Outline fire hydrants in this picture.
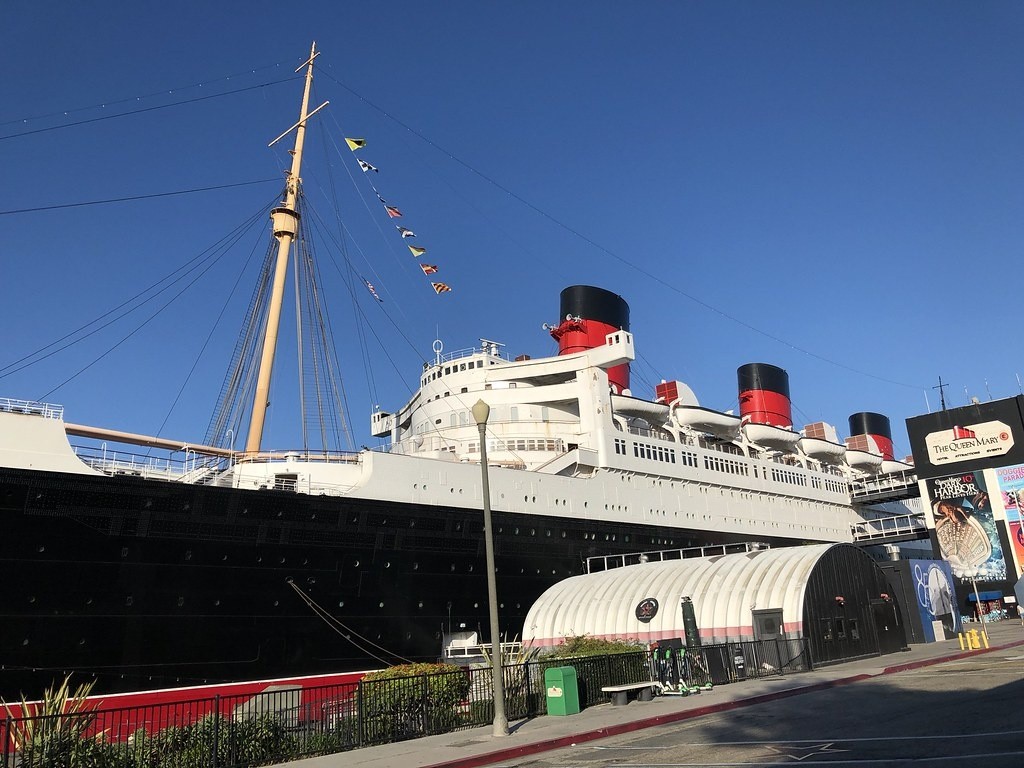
[966,628,981,649]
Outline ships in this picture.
[0,40,928,704]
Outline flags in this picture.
[357,160,378,173]
[374,196,451,295]
[344,138,366,151]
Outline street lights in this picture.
[956,566,990,639]
[471,400,510,738]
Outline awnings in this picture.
[969,591,1002,601]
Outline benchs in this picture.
[601,680,660,707]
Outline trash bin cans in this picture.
[545,666,580,716]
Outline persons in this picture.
[936,500,973,531]
[1017,605,1024,626]
